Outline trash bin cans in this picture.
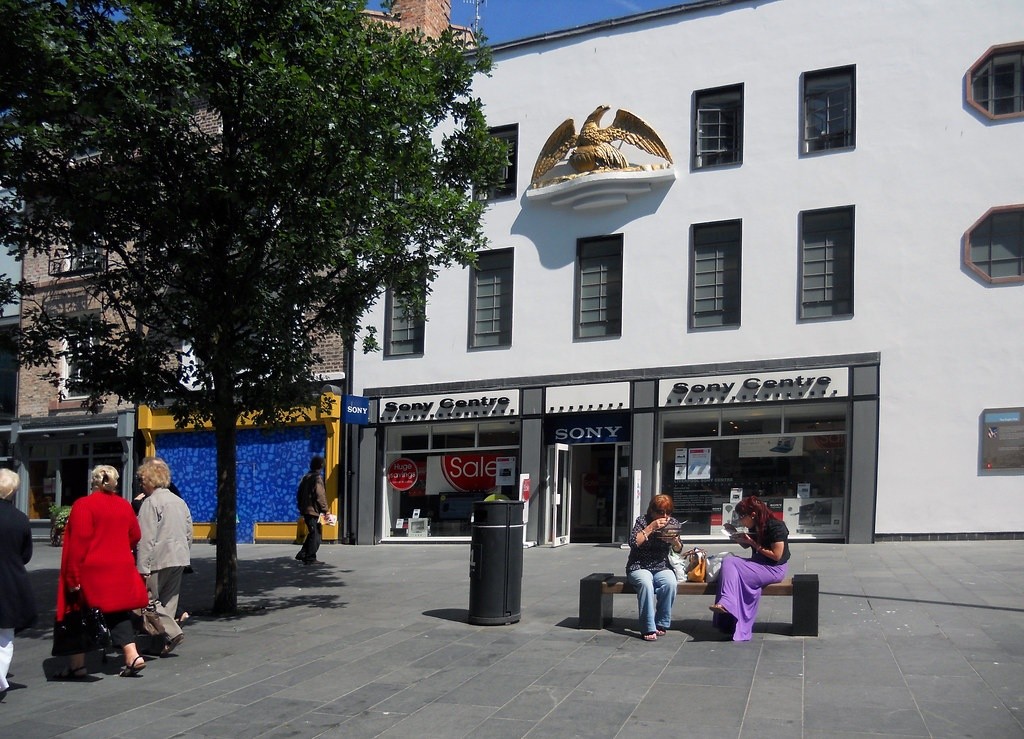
[468,500,523,625]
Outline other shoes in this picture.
[159,634,183,657]
[295,551,326,566]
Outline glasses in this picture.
[653,509,673,515]
[738,513,750,519]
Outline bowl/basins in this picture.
[733,533,756,540]
[661,524,682,537]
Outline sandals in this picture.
[175,611,191,630]
[118,656,146,678]
[656,625,666,636]
[52,666,88,681]
[641,632,658,641]
[709,603,729,614]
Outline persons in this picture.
[295,456,328,565]
[0,468,33,701]
[626,495,683,641]
[56,457,193,677]
[709,496,791,641]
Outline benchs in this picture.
[579,573,819,636]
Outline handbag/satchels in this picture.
[667,545,730,584]
[51,591,114,657]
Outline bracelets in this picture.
[642,530,648,541]
